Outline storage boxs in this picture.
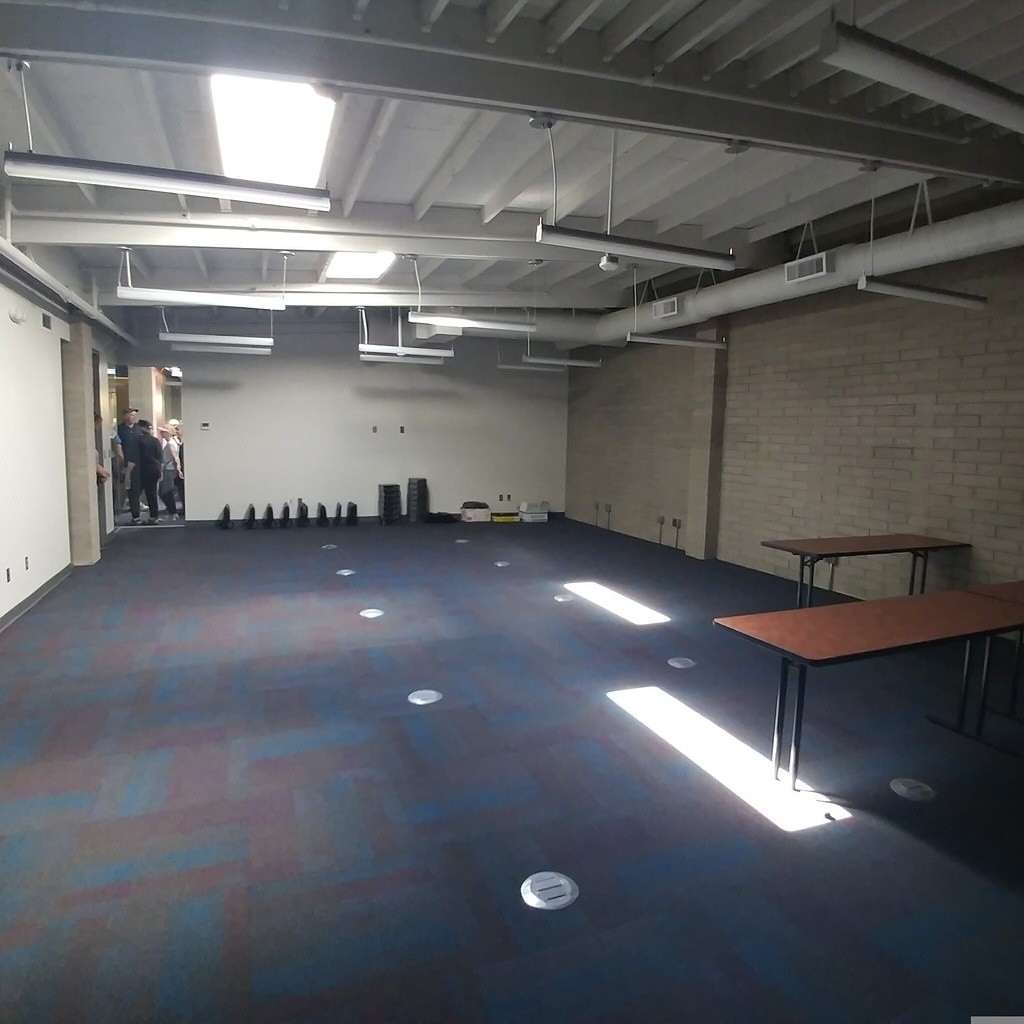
[492,511,520,522]
[519,501,550,523]
[461,508,491,522]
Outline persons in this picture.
[158,423,184,521]
[168,419,185,516]
[110,416,124,513]
[124,420,163,524]
[94,411,110,485]
[117,407,150,512]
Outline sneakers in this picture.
[148,517,166,525]
[131,518,146,526]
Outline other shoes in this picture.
[164,514,179,521]
[140,502,149,511]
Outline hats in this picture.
[158,427,173,434]
[122,407,140,415]
[168,419,179,425]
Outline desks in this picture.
[760,533,972,609]
[712,580,1024,791]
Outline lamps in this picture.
[3,60,340,211]
[820,0,1024,136]
[599,127,624,270]
[357,254,604,373]
[111,248,296,355]
[527,117,749,271]
[857,169,991,310]
[626,263,729,351]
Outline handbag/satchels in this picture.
[148,459,164,482]
